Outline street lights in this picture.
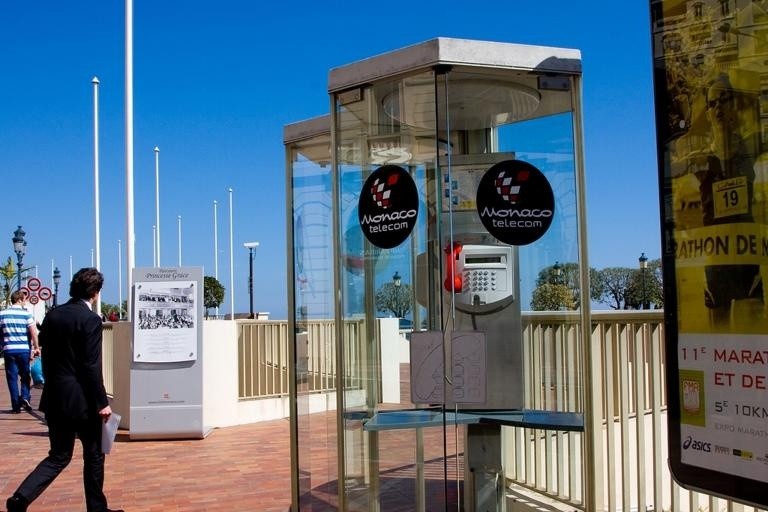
[53,267,61,307]
[638,252,649,310]
[11,225,27,290]
[552,261,561,311]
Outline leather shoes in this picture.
[6,496,26,512]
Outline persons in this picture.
[669,71,768,329]
[0,290,41,414]
[6,267,124,512]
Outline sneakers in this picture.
[14,399,31,413]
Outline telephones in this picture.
[445,241,515,314]
